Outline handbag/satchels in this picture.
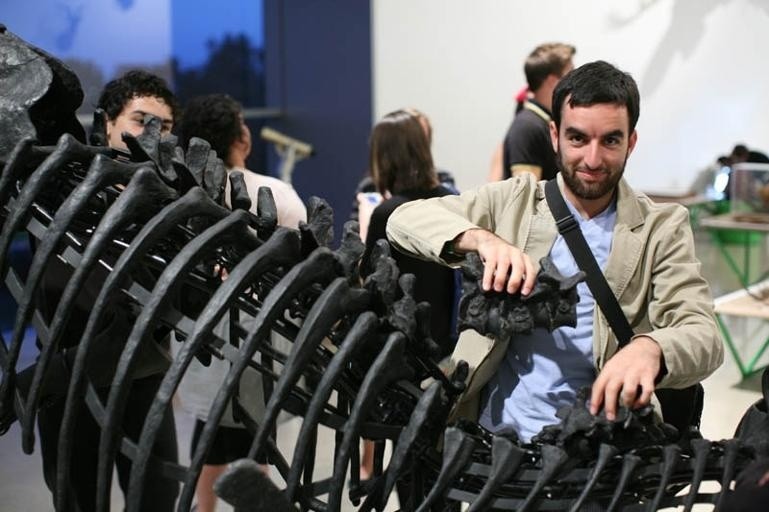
[654,382,704,429]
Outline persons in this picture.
[386,61,724,512]
[171,94,308,512]
[489,85,529,183]
[346,111,455,489]
[358,109,462,512]
[503,42,576,182]
[37,71,228,512]
